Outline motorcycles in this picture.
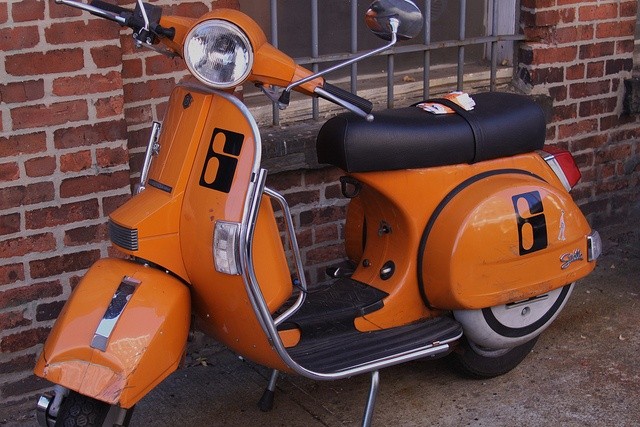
[33,0,602,427]
